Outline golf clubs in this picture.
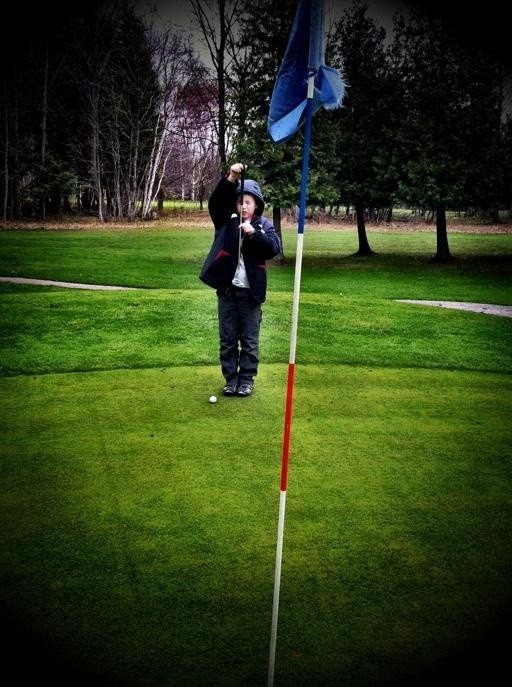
[232,164,245,288]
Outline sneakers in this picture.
[237,384,254,396]
[223,384,238,395]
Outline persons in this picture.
[199,162,283,396]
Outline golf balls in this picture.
[209,396,217,402]
